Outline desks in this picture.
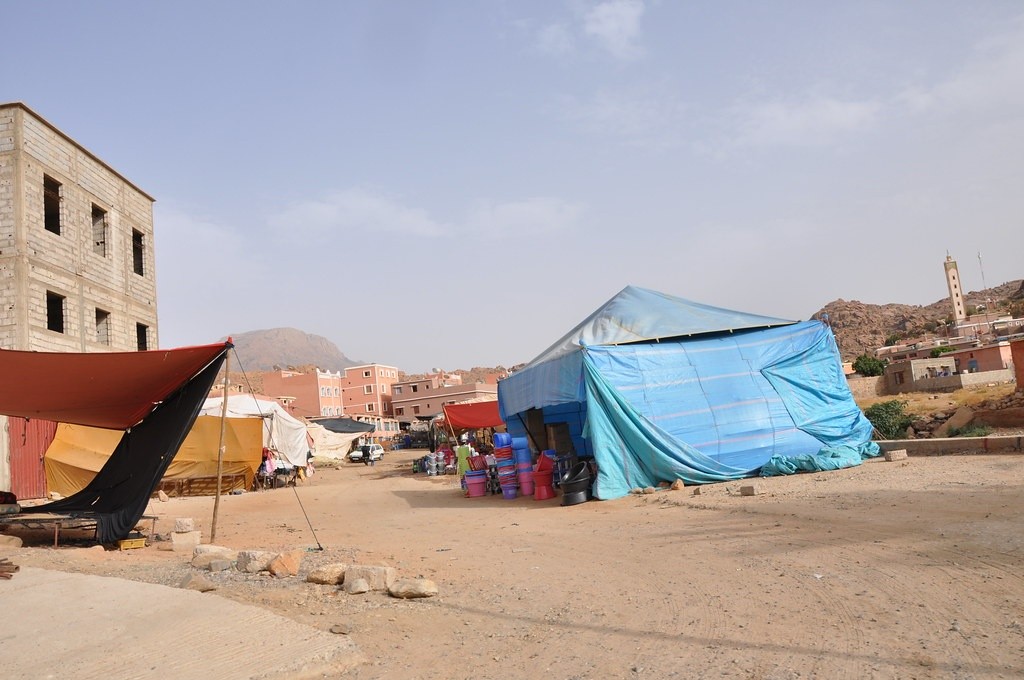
[150,478,181,497]
[186,473,246,496]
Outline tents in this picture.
[197,395,307,466]
[430,285,879,500]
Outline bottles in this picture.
[0,504,23,515]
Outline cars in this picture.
[349,443,386,463]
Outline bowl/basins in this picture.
[536,451,552,472]
[529,471,553,486]
[534,486,556,501]
[560,489,593,506]
[560,461,589,482]
[557,476,590,494]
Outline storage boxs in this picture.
[524,410,572,454]
[115,538,147,550]
[475,426,493,447]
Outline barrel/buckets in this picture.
[458,433,534,499]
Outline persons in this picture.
[485,448,497,477]
[454,434,463,445]
[370,455,374,465]
[361,443,370,466]
[489,427,496,444]
